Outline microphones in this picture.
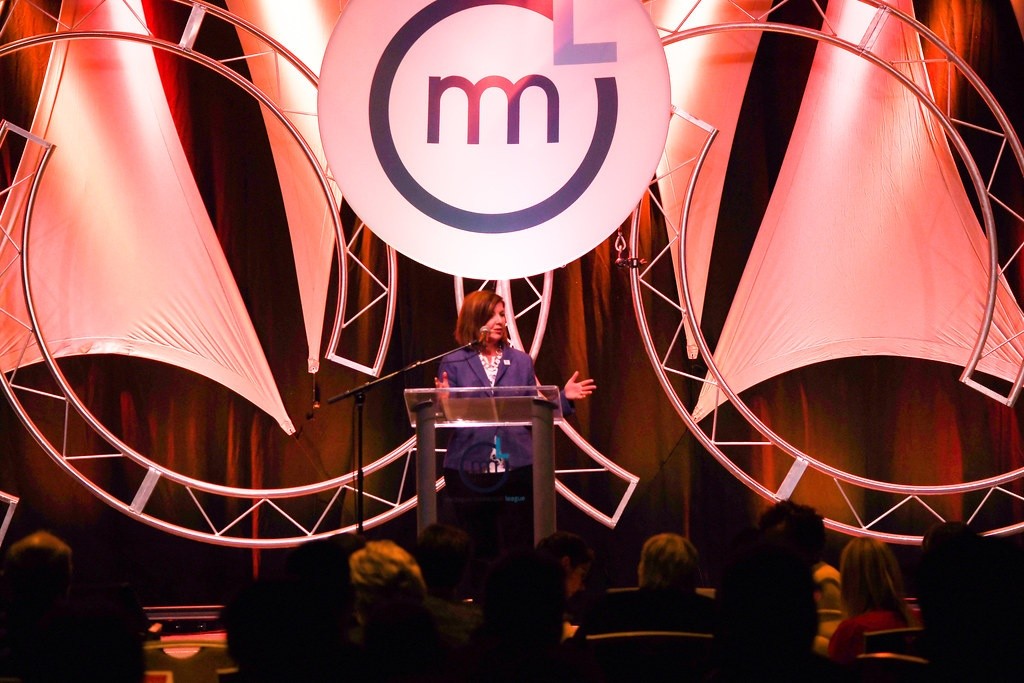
[479,326,491,347]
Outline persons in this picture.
[0,500,1024,683]
[434,290,596,601]
